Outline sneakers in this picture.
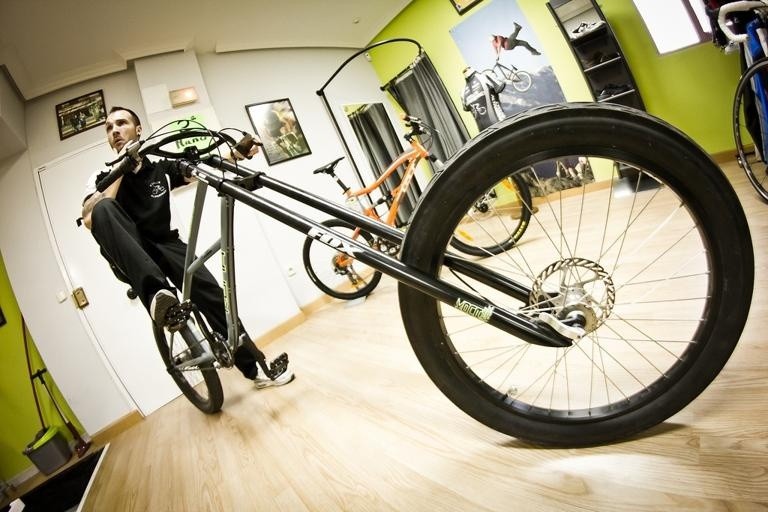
[577,48,622,73]
[253,358,296,390]
[566,19,607,43]
[514,22,522,30]
[531,50,541,55]
[594,79,635,104]
[148,287,183,335]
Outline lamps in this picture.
[169,86,200,108]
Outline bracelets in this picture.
[230,150,244,161]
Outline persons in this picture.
[459,67,507,133]
[81,104,295,390]
[79,112,86,128]
[487,21,542,62]
[257,105,303,160]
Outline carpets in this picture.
[0,440,112,512]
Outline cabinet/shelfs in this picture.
[546,0,664,192]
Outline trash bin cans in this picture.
[22,426,73,476]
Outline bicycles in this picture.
[483,53,537,100]
[74,124,756,454]
[717,0,767,211]
[302,109,537,305]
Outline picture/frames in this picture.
[245,98,312,167]
[450,0,483,16]
[55,89,108,141]
